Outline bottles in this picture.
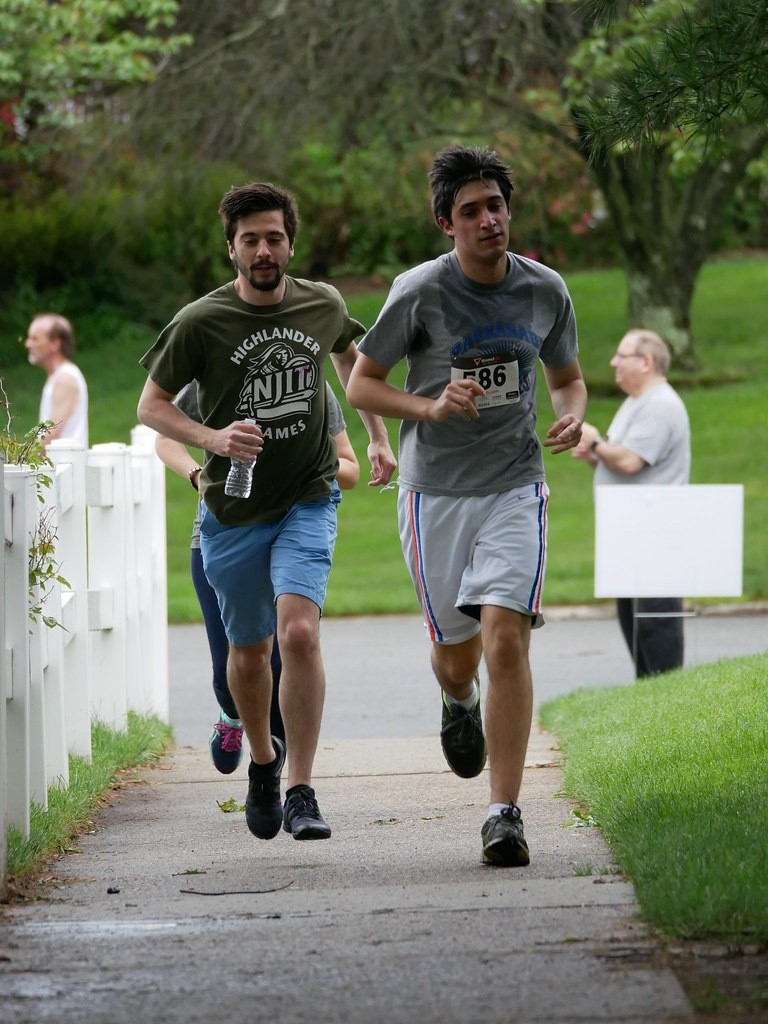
[224,417,258,499]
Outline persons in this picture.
[570,326,692,682]
[346,142,590,870]
[133,180,398,843]
[153,376,360,775]
[24,312,90,465]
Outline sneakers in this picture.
[209,710,244,774]
[283,784,331,839]
[245,737,285,840]
[481,792,530,867]
[441,669,488,778]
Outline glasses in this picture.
[615,351,642,361]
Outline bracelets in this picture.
[188,467,204,491]
[590,436,605,452]
